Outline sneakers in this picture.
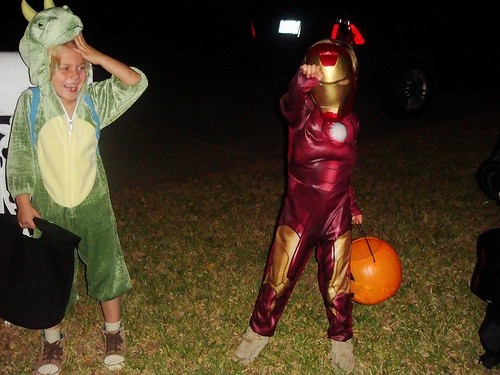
[37,332,69,375]
[101,323,128,372]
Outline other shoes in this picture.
[328,337,356,375]
[233,325,271,366]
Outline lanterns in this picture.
[348,237,401,305]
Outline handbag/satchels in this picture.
[0,212,83,330]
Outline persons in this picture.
[6,0,150,375]
[233,20,366,375]
